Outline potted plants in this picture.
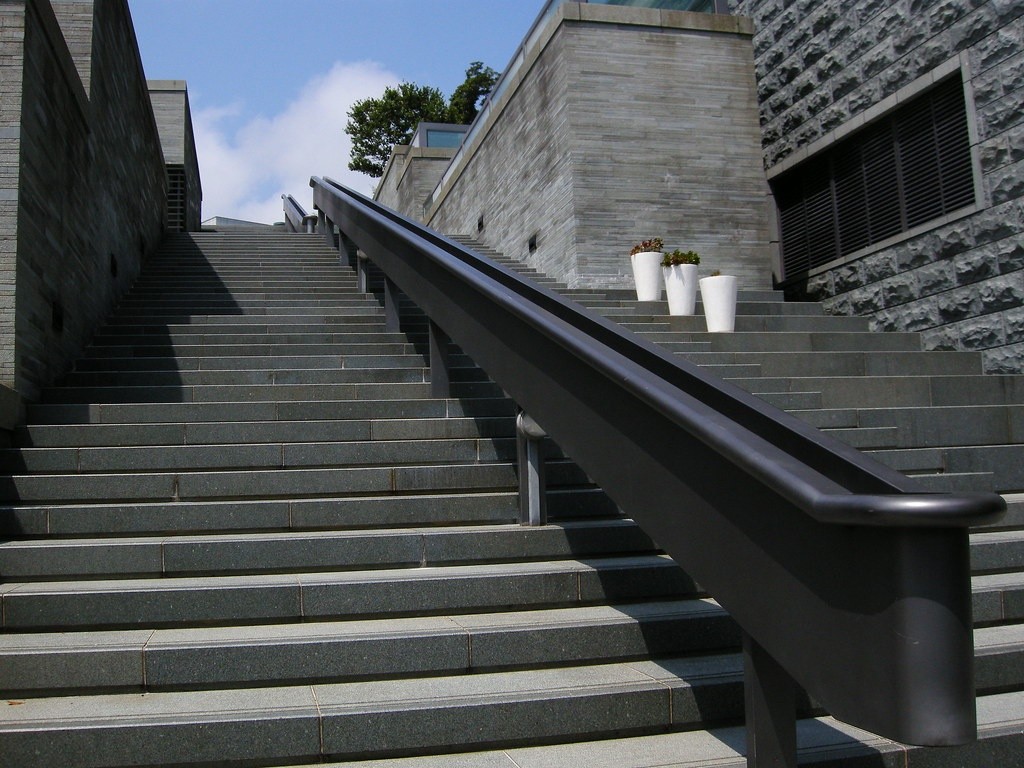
[698,270,739,333]
[629,236,666,301]
[660,248,699,316]
